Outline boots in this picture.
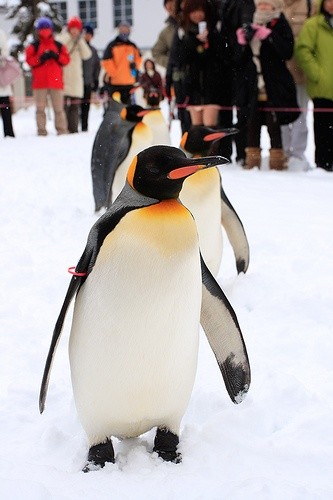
[270,149,283,170]
[243,148,261,169]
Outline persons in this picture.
[230,0,304,171]
[54,16,92,133]
[79,23,101,132]
[0,30,21,138]
[295,0,333,172]
[281,0,321,172]
[25,17,71,136]
[101,21,142,105]
[218,0,256,166]
[165,0,232,125]
[151,0,185,68]
[140,59,165,108]
[96,72,113,112]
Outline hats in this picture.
[83,26,93,35]
[255,0,280,9]
[68,17,82,33]
[36,18,52,29]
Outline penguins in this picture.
[39,144,251,473]
[105,104,161,210]
[179,124,250,280]
[141,88,171,149]
[91,82,144,213]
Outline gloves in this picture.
[236,28,246,45]
[251,24,271,40]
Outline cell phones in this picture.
[199,22,207,35]
